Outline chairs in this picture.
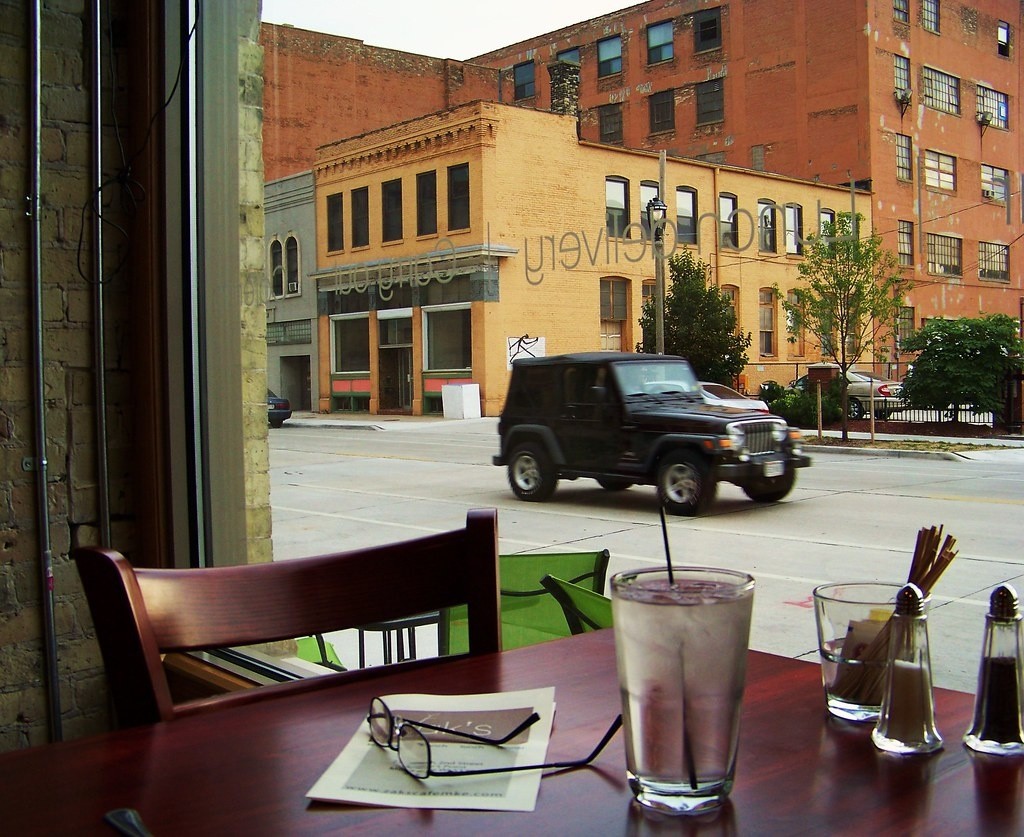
[291,629,346,676]
[73,506,504,727]
[539,572,615,637]
[437,545,610,657]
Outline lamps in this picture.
[980,110,993,135]
[900,88,912,116]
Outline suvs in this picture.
[493,353,813,516]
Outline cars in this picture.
[783,371,911,418]
[646,380,768,414]
[267,387,292,427]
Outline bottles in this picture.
[868,583,947,754]
[963,583,1024,757]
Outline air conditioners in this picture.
[288,281,298,294]
[985,189,995,199]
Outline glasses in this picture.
[368,697,623,779]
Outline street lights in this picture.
[647,200,668,355]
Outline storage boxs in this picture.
[441,382,481,420]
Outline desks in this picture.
[0,621,1024,836]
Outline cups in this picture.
[811,579,933,724]
[609,566,755,816]
